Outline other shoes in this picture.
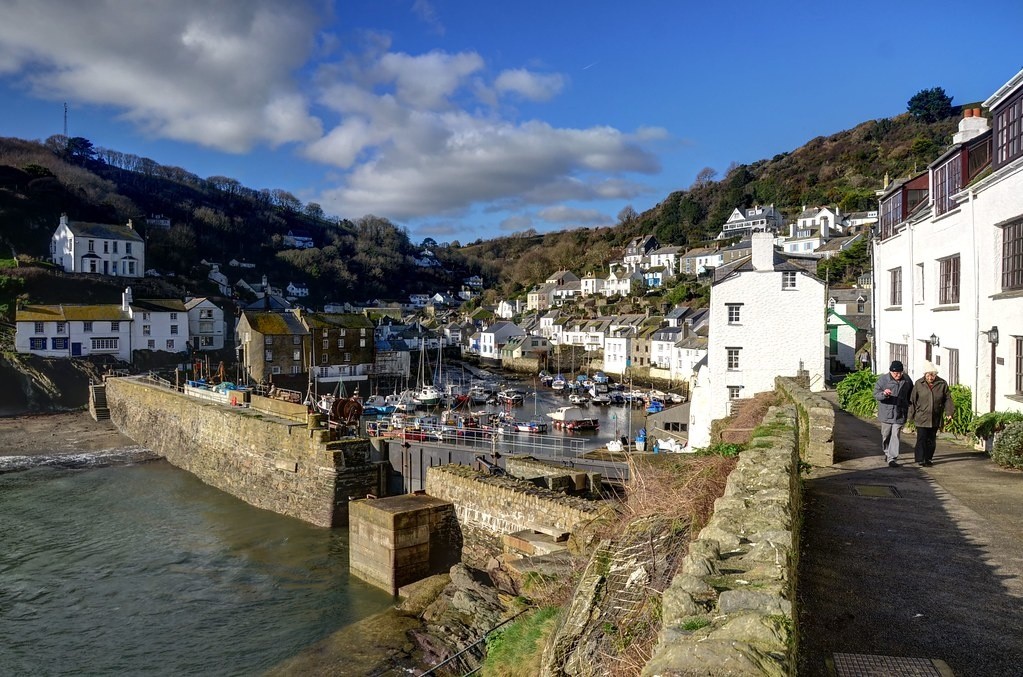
[926,459,934,467]
[917,461,925,465]
[888,461,899,467]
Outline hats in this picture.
[923,362,938,374]
[890,361,903,371]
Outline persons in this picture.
[907,362,953,466]
[859,349,869,370]
[873,361,914,467]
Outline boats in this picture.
[184,337,686,439]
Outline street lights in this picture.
[981,326,999,413]
